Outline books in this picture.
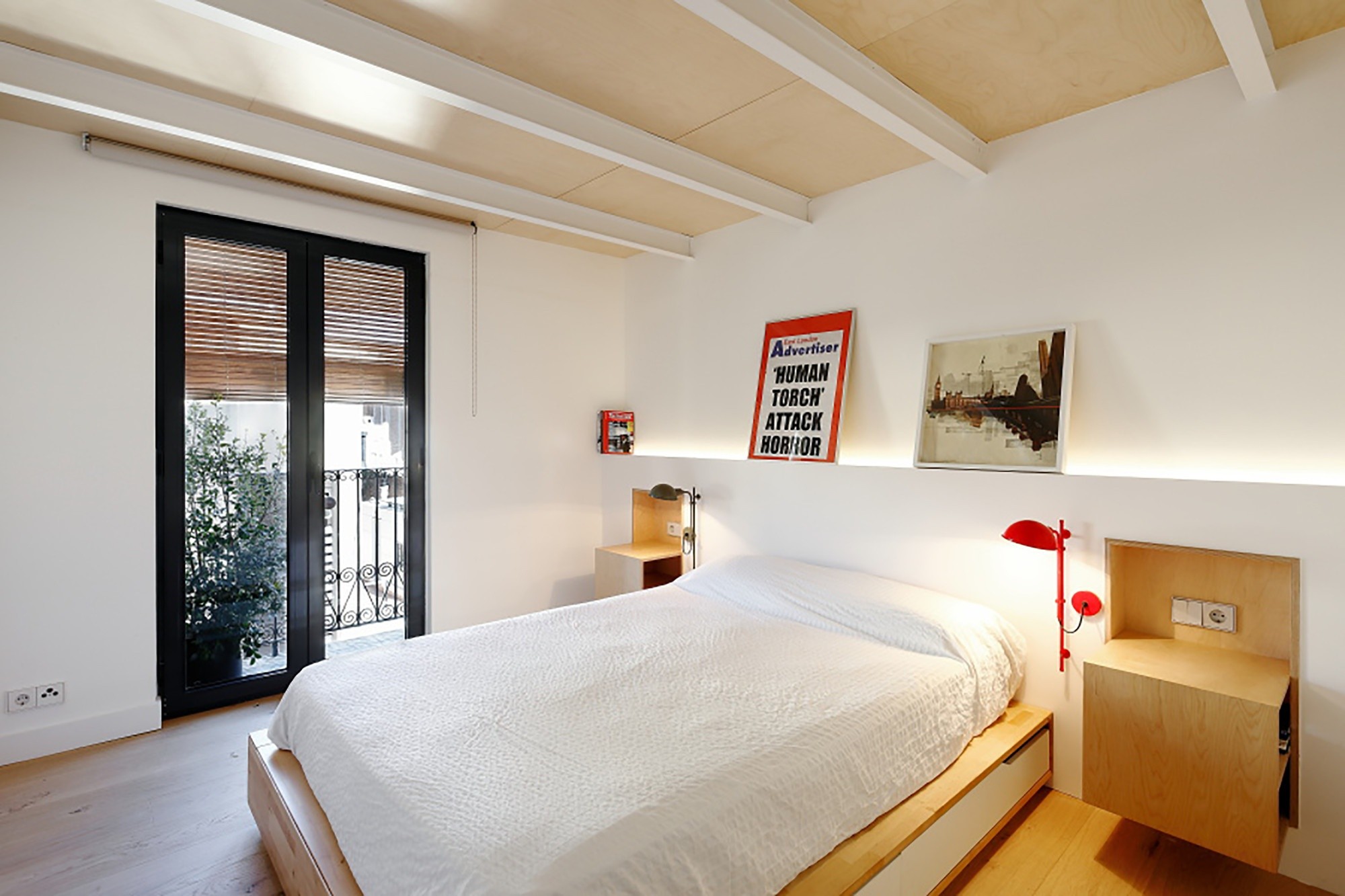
[601,410,635,454]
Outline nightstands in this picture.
[594,542,682,600]
[1085,626,1295,876]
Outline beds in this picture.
[246,551,1054,895]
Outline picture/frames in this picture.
[913,320,1078,474]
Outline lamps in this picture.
[650,484,701,569]
[1003,517,1102,672]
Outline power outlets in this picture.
[667,522,679,536]
[1201,601,1238,634]
[6,682,66,713]
